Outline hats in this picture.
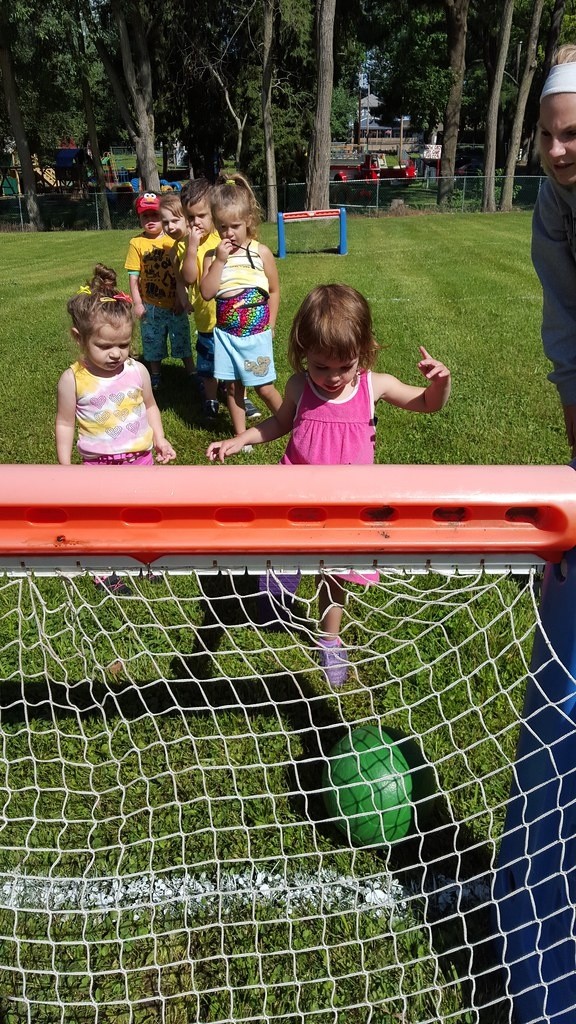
[136,193,163,214]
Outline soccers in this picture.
[320,724,436,849]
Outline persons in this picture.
[124,173,291,455]
[208,286,451,686]
[55,263,176,601]
[531,43,575,470]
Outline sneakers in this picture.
[319,641,349,686]
[204,401,219,422]
[259,570,300,597]
[244,399,262,419]
[146,574,164,584]
[190,373,200,382]
[94,574,133,597]
[151,374,161,389]
